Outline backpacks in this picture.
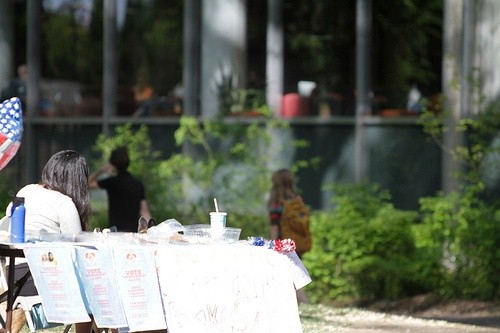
[280,195,312,253]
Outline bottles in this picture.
[11,197,25,242]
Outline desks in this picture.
[0,235,76,333]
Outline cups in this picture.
[209,212,227,228]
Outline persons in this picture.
[267,169,304,260]
[41,252,56,265]
[88,148,151,232]
[5,149,93,333]
